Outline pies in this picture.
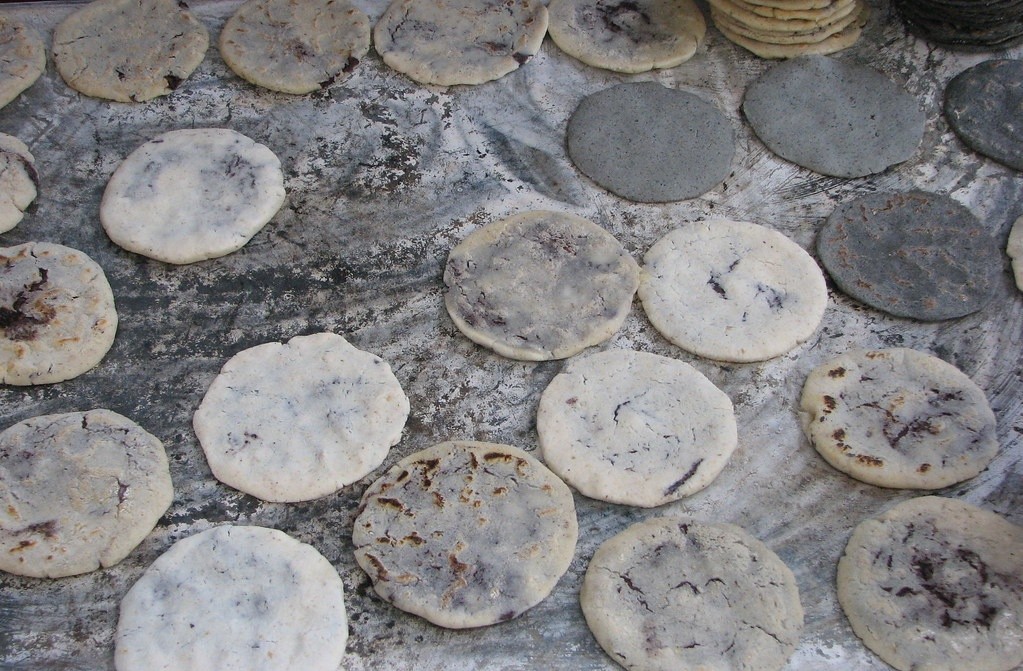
[0,0,1023,671]
